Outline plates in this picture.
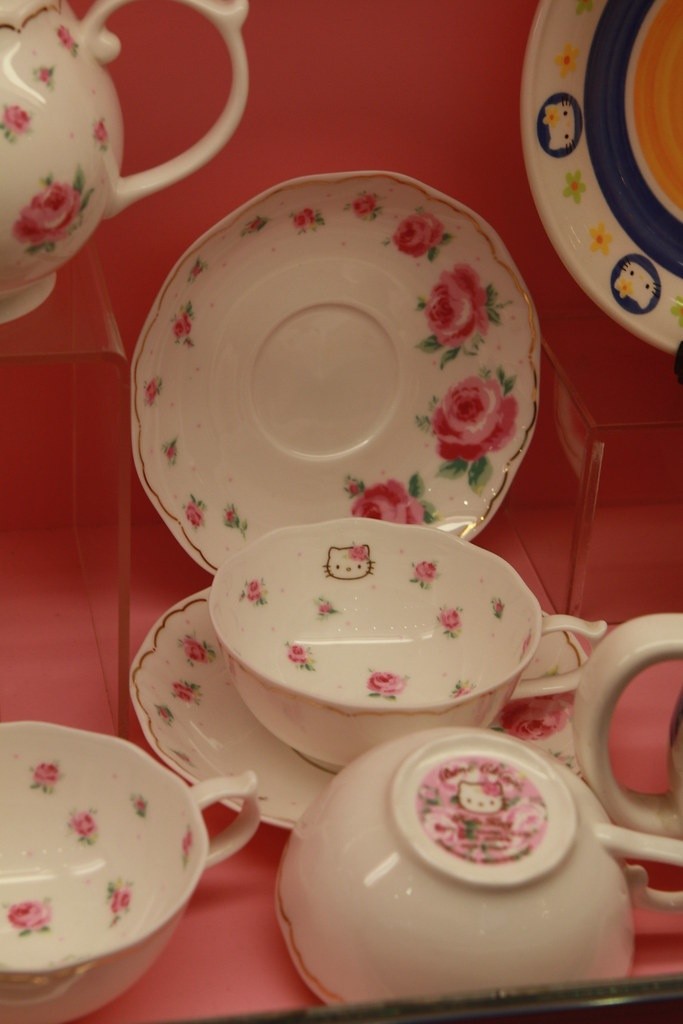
[129,169,543,578]
[521,0,682,360]
[129,585,589,829]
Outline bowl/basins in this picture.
[273,725,683,1004]
[0,719,262,1024]
[209,517,608,774]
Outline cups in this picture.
[571,612,683,836]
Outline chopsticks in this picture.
[162,973,682,1024]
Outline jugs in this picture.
[1,0,250,325]
